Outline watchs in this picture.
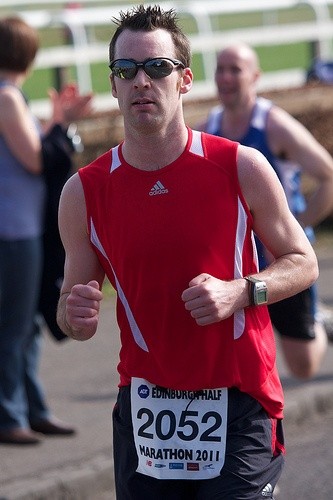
[243,275,269,308]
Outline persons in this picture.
[0,16,98,446]
[196,42,333,381]
[56,5,319,500]
[305,40,333,87]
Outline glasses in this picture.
[109,56,187,82]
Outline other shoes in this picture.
[35,414,74,434]
[0,428,40,445]
